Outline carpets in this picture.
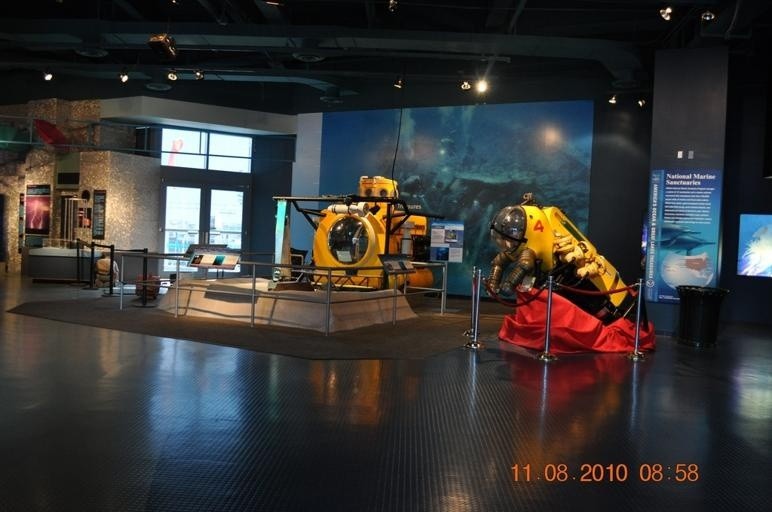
[6,292,518,363]
